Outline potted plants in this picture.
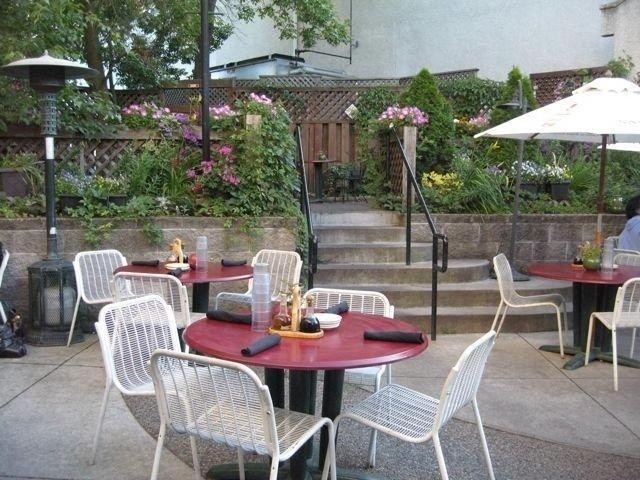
[96,176,130,214]
[0,151,40,198]
[56,173,86,215]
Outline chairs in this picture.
[0,249,10,325]
[342,162,368,203]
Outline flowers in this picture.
[483,166,507,184]
[546,153,573,181]
[511,159,539,181]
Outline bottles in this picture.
[195,236,209,274]
[299,297,319,332]
[167,244,178,263]
[178,243,188,263]
[273,294,291,329]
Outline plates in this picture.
[314,313,342,330]
[165,263,191,271]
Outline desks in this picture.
[306,159,338,204]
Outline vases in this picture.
[519,182,538,199]
[550,181,570,201]
[492,183,508,202]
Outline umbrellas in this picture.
[473,76,640,247]
[597,143,640,152]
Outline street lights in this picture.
[492,79,536,284]
[1,49,101,347]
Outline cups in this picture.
[250,263,270,331]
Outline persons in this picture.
[616,196,640,252]
